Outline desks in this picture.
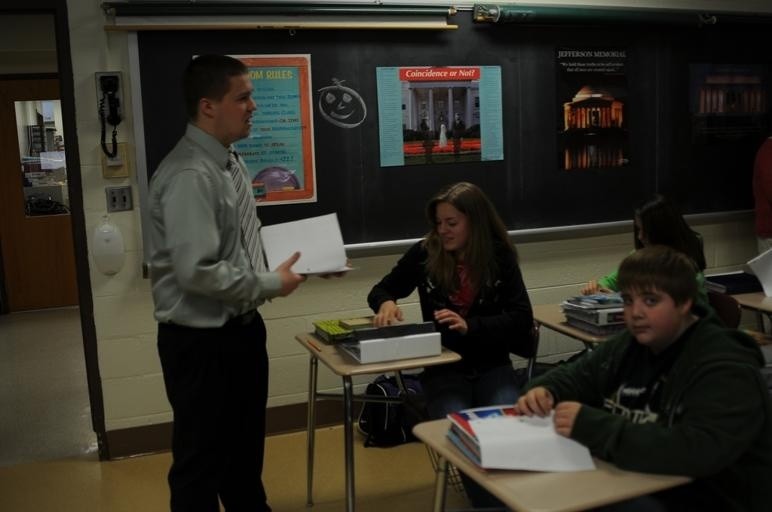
[21,178,69,209]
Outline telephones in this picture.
[95,70,125,126]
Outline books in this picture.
[446,403,597,474]
[258,213,357,276]
[745,247,771,297]
[311,313,445,369]
[560,72,765,170]
[559,281,630,338]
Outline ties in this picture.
[228,155,271,304]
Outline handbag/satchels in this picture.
[358,375,426,446]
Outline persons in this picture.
[143,53,351,511]
[580,192,711,301]
[510,240,770,512]
[367,182,539,442]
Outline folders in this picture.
[560,308,626,326]
[340,333,441,364]
